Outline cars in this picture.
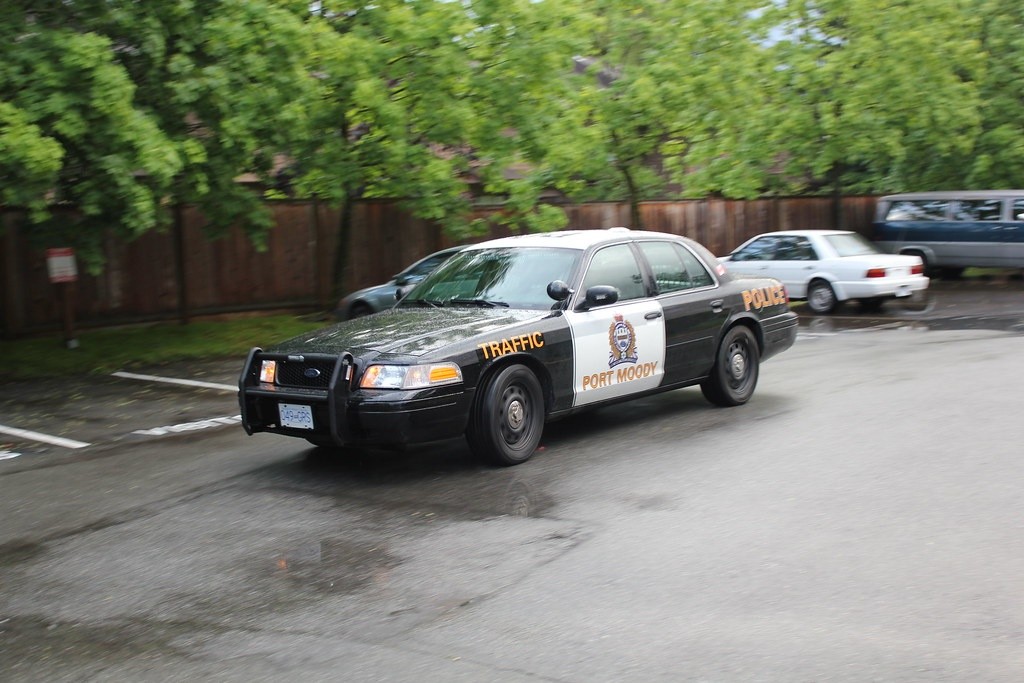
[714,228,931,316]
[236,226,799,468]
[336,242,476,323]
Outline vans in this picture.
[867,188,1024,279]
